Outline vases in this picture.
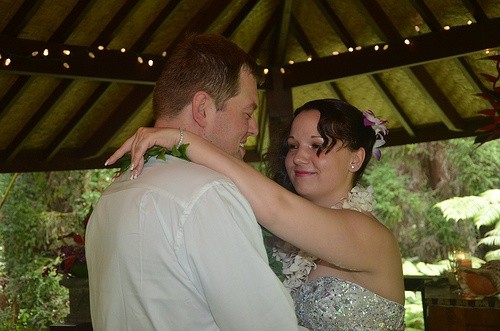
[59,279,91,324]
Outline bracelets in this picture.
[175,126,184,152]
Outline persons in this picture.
[104,99,406,331]
[85,34,309,331]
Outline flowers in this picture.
[267,186,377,293]
[33,205,93,287]
[361,109,389,161]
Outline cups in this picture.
[448,251,473,295]
